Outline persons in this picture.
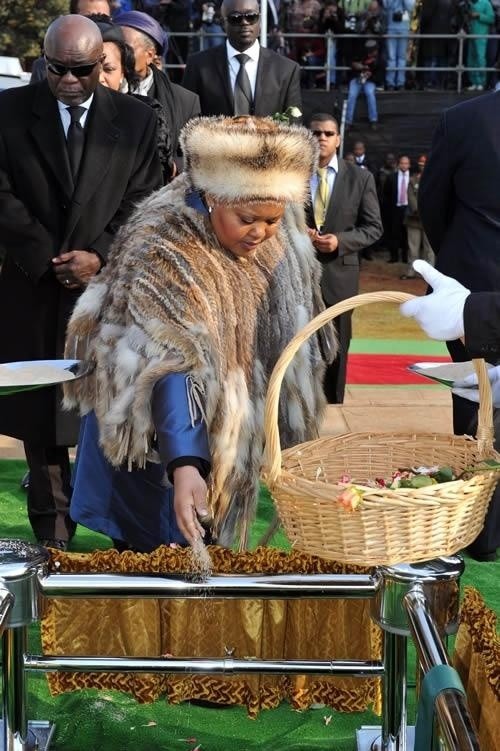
[2,14,165,552]
[298,112,383,408]
[4,1,498,490]
[62,116,339,554]
[398,260,499,560]
[417,94,499,440]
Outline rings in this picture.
[66,280,69,284]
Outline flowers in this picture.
[332,454,500,514]
[273,104,304,124]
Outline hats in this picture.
[180,115,320,205]
[116,6,170,55]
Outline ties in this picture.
[234,54,253,115]
[313,167,330,232]
[66,107,86,186]
[400,174,406,203]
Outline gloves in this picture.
[400,259,471,342]
[451,365,499,407]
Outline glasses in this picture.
[43,53,98,77]
[226,13,259,25]
[312,130,336,136]
